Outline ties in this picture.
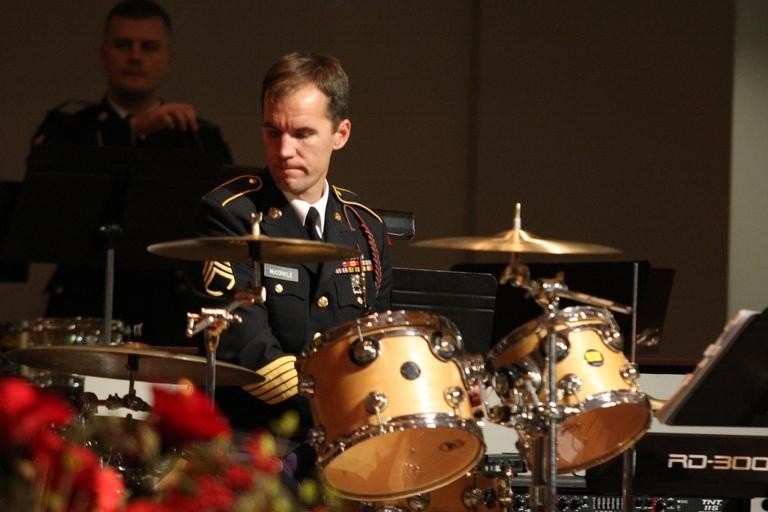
[306,207,320,241]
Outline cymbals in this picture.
[147,235,362,262]
[8,341,265,386]
[409,227,622,255]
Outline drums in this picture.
[375,470,511,511]
[295,310,486,502]
[486,307,652,476]
[1,316,124,391]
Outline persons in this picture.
[22,4,235,359]
[188,49,396,481]
